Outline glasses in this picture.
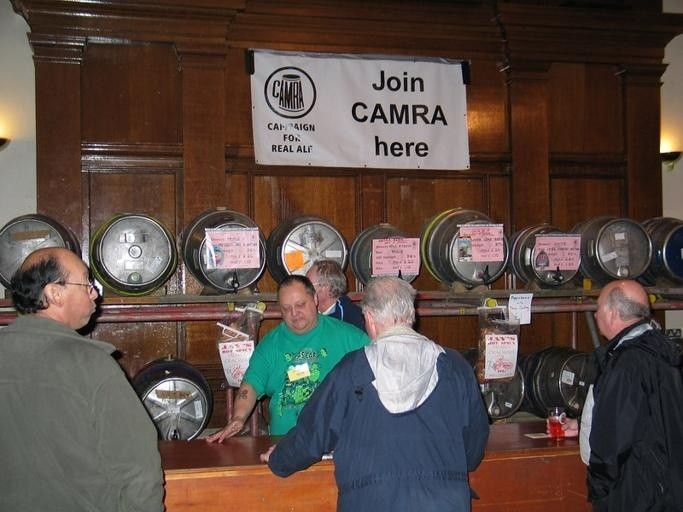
[57,280,95,295]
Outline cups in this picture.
[548,407,566,444]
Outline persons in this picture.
[258,275,491,512]
[305,260,368,335]
[205,275,370,444]
[578,278,683,512]
[0,246,167,512]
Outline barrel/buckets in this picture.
[131,358,213,442]
[639,216,682,287]
[423,208,511,286]
[90,208,178,299]
[456,346,526,420]
[351,219,424,292]
[267,214,350,294]
[182,207,267,297]
[512,223,581,288]
[580,215,655,287]
[2,208,84,300]
[525,348,599,420]
